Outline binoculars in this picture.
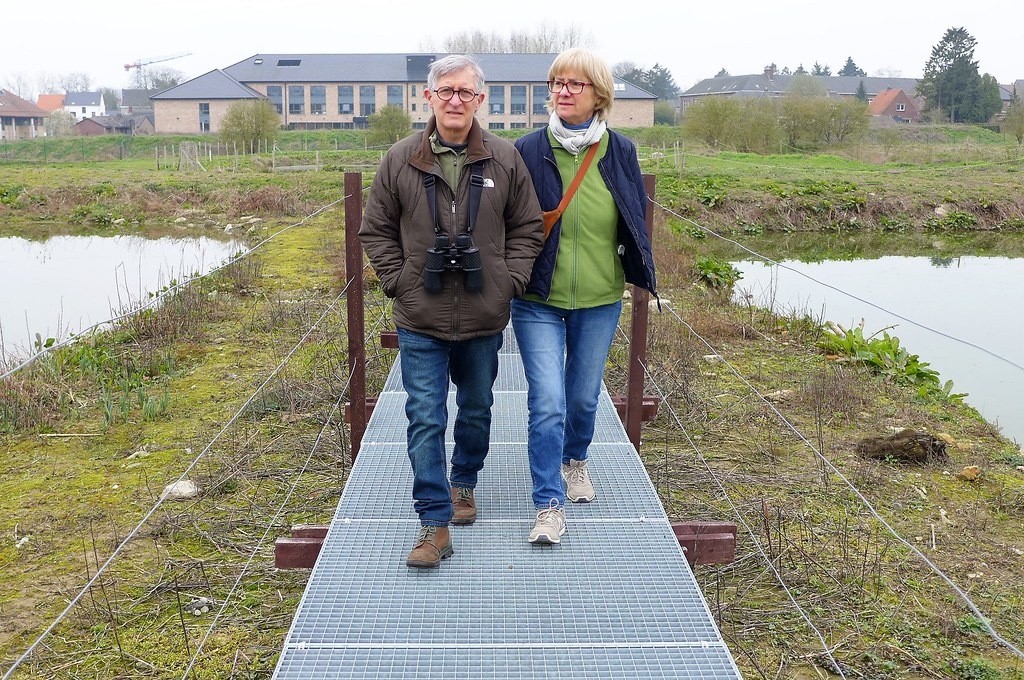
[423,232,485,293]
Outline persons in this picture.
[510,49,663,543]
[359,55,546,566]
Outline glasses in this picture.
[430,86,479,103]
[547,79,594,95]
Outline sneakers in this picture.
[528,497,566,543]
[406,526,453,567]
[451,487,477,523]
[561,458,595,502]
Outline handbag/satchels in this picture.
[542,209,561,241]
